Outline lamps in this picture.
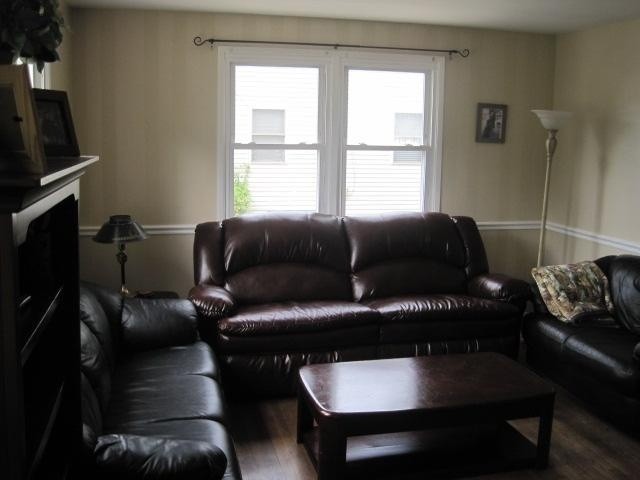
[531,109,580,267]
[93,215,151,297]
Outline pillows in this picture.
[608,255,640,335]
[79,286,115,436]
[223,212,467,302]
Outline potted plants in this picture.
[0,0,65,74]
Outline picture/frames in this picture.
[476,103,507,143]
[33,88,80,157]
[0,64,48,174]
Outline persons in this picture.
[483,111,500,138]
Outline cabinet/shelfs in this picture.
[0,170,84,480]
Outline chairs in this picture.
[521,255,640,441]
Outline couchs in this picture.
[79,280,242,480]
[187,212,531,398]
